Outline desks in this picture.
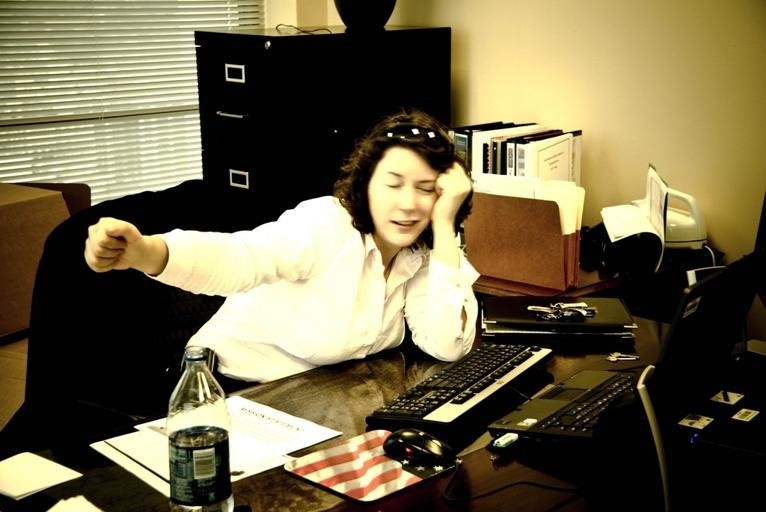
[0,295,674,511]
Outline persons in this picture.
[82,109,481,407]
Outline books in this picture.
[445,119,583,188]
[482,295,638,343]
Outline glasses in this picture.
[368,124,451,150]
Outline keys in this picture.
[606,356,636,362]
[610,351,639,360]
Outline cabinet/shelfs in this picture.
[193,24,453,206]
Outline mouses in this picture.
[382,429,455,467]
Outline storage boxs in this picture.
[0,184,72,340]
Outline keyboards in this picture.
[364,343,554,431]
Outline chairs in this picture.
[34,180,260,469]
[11,183,91,213]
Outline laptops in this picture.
[488,247,761,456]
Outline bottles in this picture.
[168,347,234,512]
